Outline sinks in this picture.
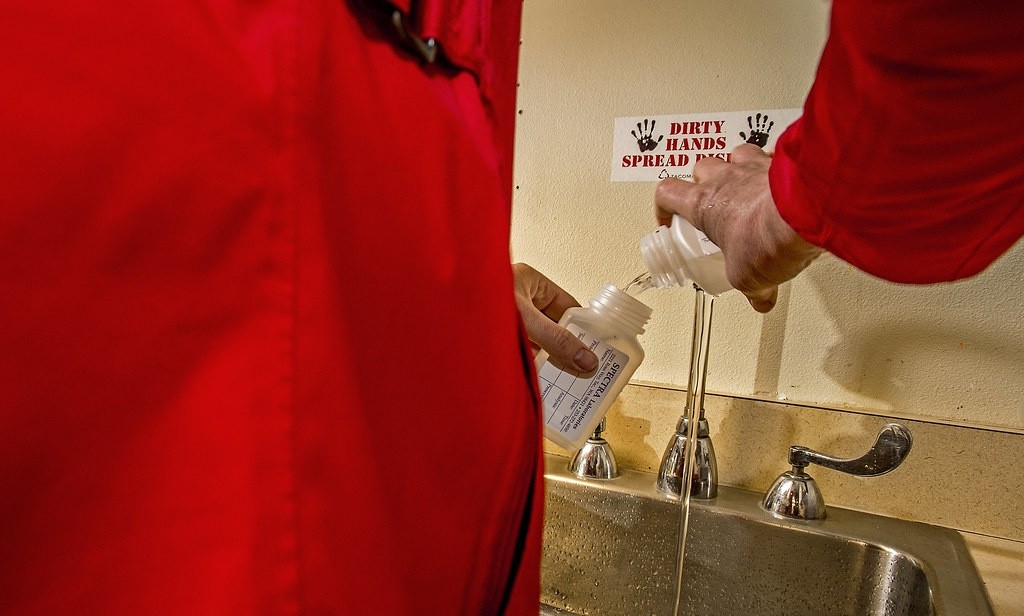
[541,450,995,616]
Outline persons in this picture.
[0,0,1024,616]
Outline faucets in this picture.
[656,283,717,501]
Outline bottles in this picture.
[534,283,652,450]
[640,214,735,296]
